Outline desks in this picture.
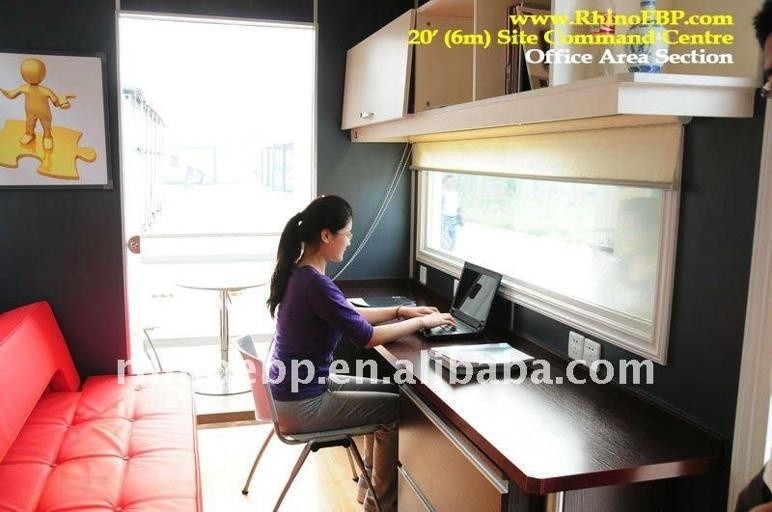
[165,261,271,397]
[336,278,724,512]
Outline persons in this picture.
[264,193,458,511]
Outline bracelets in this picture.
[396,304,402,321]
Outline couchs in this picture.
[0,300,205,512]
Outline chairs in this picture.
[234,334,389,512]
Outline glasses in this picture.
[338,230,353,240]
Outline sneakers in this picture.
[356,486,396,512]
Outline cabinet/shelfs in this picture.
[340,0,758,142]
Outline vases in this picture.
[621,0,671,74]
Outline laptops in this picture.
[415,260,502,341]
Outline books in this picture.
[427,342,536,375]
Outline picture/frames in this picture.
[0,43,117,193]
